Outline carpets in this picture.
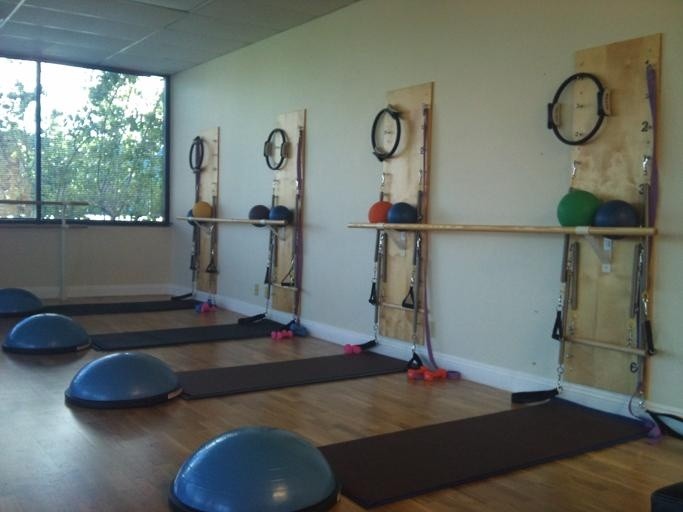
[171,349,418,401]
[86,318,291,353]
[315,396,655,510]
[649,482,683,512]
[32,298,217,317]
